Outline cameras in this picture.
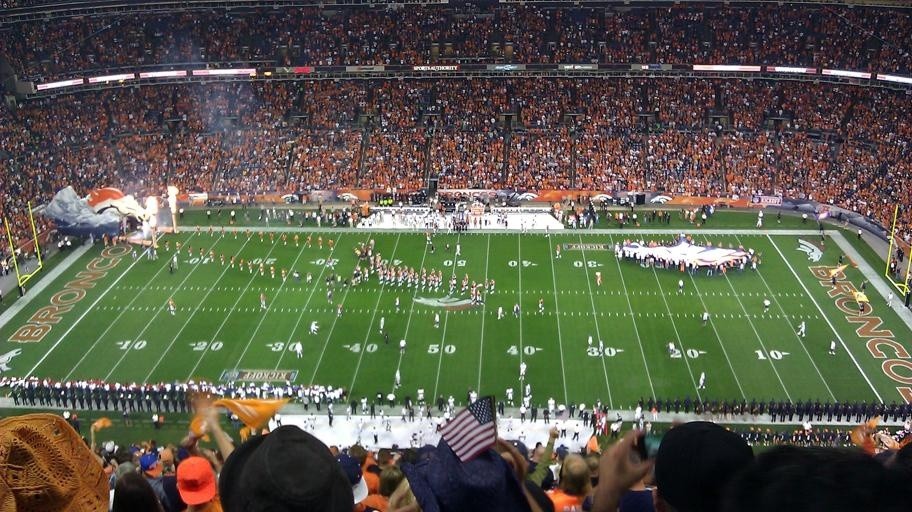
[637,434,663,460]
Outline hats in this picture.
[176,455,217,506]
[140,455,157,471]
[654,421,754,512]
[217,424,354,512]
[401,437,535,511]
[0,414,110,512]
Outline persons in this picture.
[702,310,710,325]
[311,321,319,336]
[434,312,441,329]
[797,320,806,338]
[519,361,527,380]
[828,338,837,356]
[497,306,505,320]
[394,296,401,315]
[399,336,407,355]
[762,296,771,314]
[379,315,386,334]
[512,303,521,318]
[295,340,304,359]
[666,341,676,354]
[383,332,390,345]
[587,334,593,351]
[126,1,908,308]
[1,366,911,512]
[537,297,545,316]
[1,1,128,276]
[18,280,26,298]
[167,298,177,316]
[598,340,604,358]
[857,301,865,318]
[336,303,345,319]
